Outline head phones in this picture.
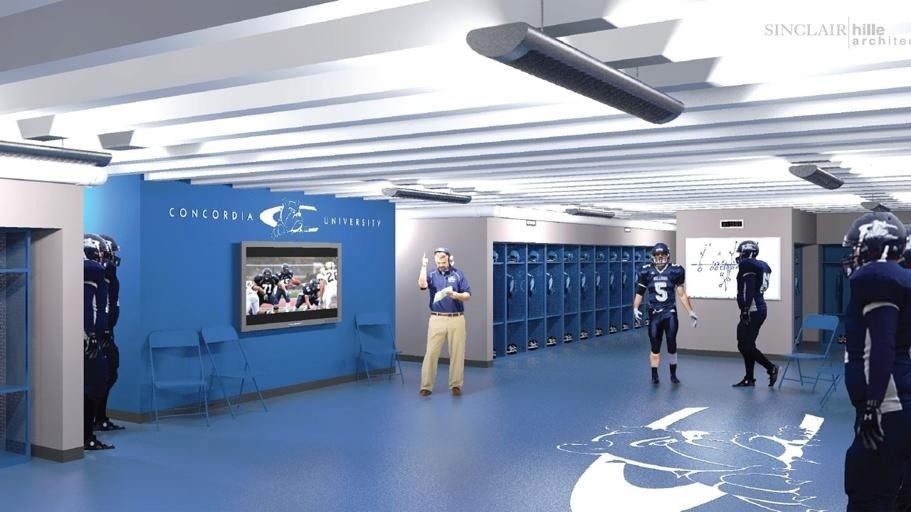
[434,248,455,267]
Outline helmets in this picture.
[737,241,759,258]
[435,248,451,257]
[842,211,906,261]
[326,262,336,270]
[309,279,320,288]
[83,233,120,260]
[493,250,650,262]
[652,243,669,255]
[255,263,289,282]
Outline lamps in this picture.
[466,1,684,125]
[383,187,471,205]
[860,195,890,212]
[566,209,615,218]
[789,164,844,190]
[0,137,112,168]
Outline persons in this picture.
[246,262,337,316]
[840,210,911,512]
[84,233,115,450]
[633,242,698,384]
[418,248,471,397]
[731,240,782,387]
[92,234,126,430]
[896,226,911,269]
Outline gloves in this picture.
[100,331,112,357]
[853,399,884,450]
[690,313,698,328]
[84,335,98,359]
[741,306,751,325]
[634,309,642,323]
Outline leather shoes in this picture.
[451,387,460,395]
[420,390,431,395]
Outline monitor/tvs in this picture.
[240,239,343,334]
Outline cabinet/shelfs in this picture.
[492,243,654,358]
[1,228,30,466]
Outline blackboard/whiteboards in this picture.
[684,236,783,302]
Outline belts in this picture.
[431,313,464,316]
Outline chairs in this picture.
[816,367,844,409]
[354,313,405,387]
[201,325,268,421]
[148,330,211,432]
[776,314,839,394]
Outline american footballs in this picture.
[291,279,301,284]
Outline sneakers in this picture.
[84,439,115,450]
[92,417,125,431]
[493,324,629,357]
[652,376,658,383]
[670,375,680,384]
[634,320,649,328]
[767,365,779,386]
[732,376,755,387]
